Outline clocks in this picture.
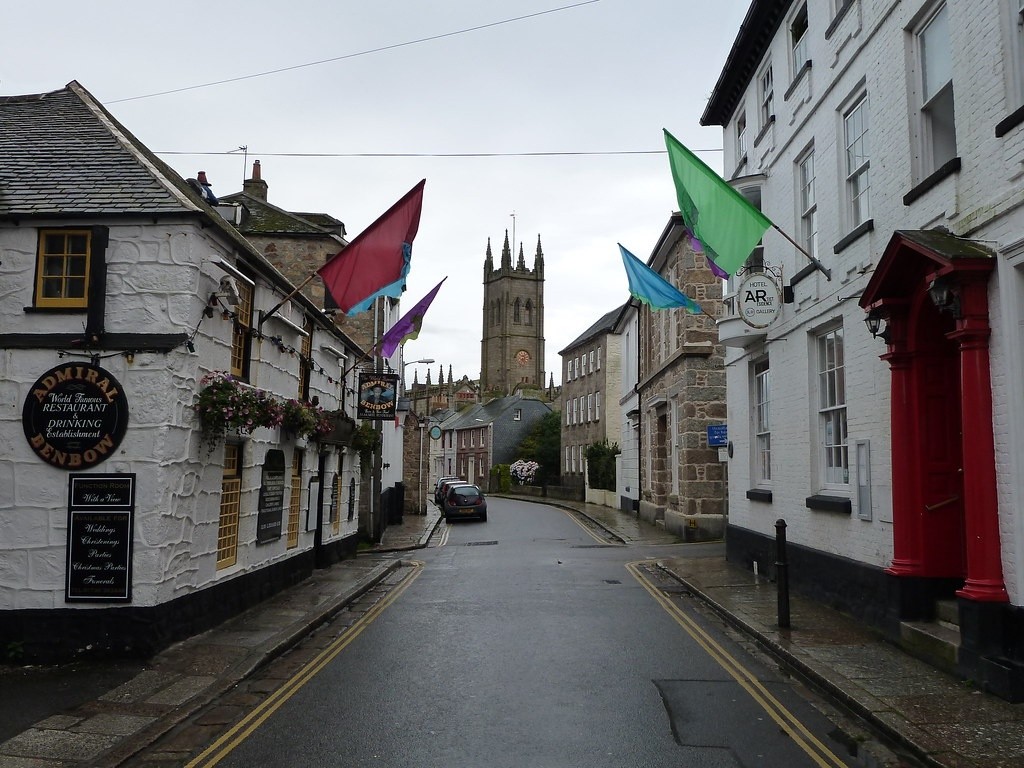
[514,349,532,366]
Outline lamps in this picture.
[927,270,961,319]
[863,304,890,344]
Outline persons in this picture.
[367,384,390,405]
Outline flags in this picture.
[373,276,448,358]
[662,127,773,280]
[616,242,702,315]
[313,178,426,317]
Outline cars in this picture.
[434,477,468,505]
[444,484,487,524]
